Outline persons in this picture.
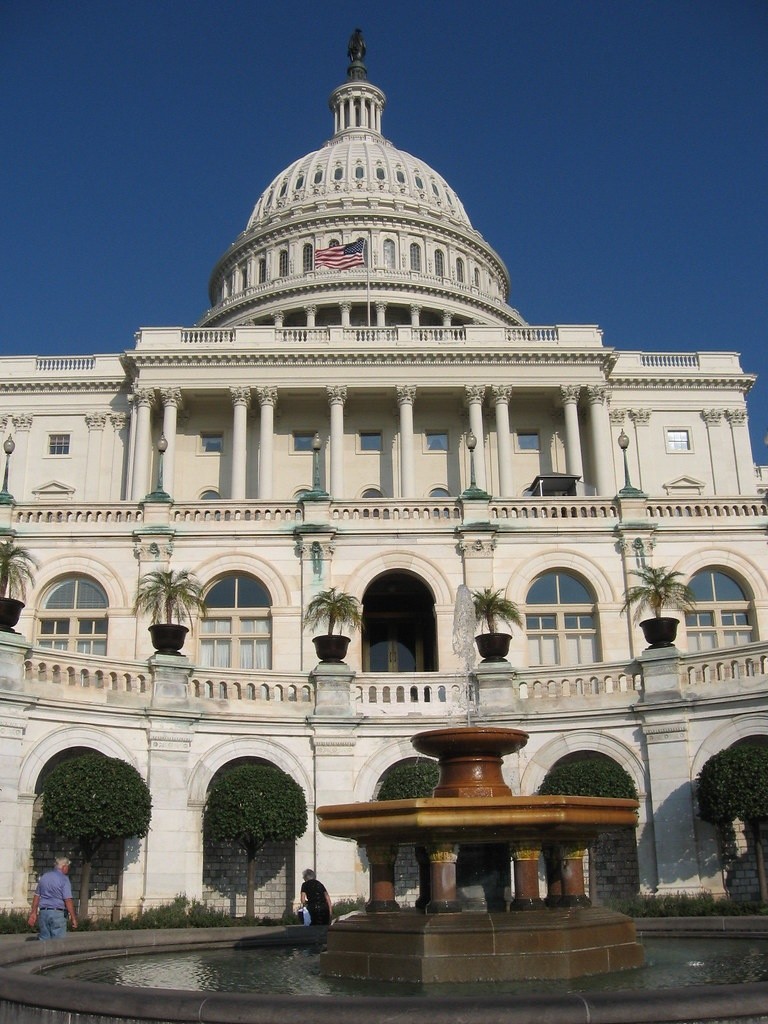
[347,27,366,62]
[300,869,333,925]
[27,856,78,941]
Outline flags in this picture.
[315,238,364,269]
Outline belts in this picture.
[40,908,63,910]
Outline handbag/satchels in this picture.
[298,901,311,924]
